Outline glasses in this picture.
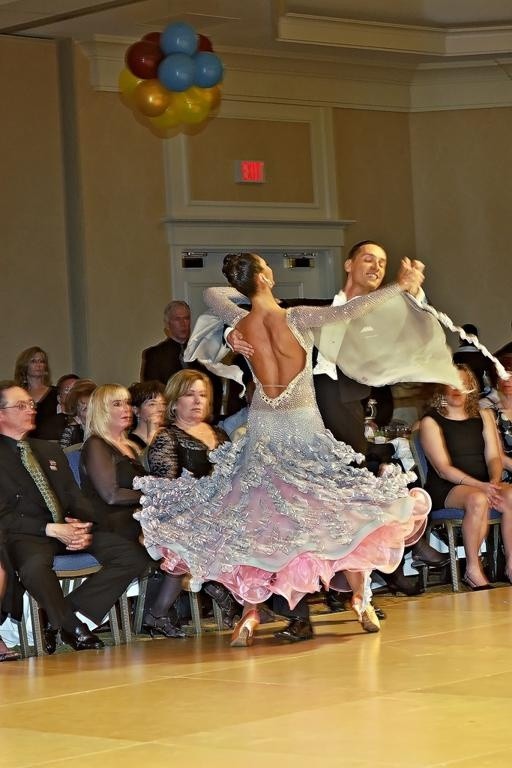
[64,387,71,392]
[1,400,37,410]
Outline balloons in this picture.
[116,20,226,141]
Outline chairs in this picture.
[409,429,503,592]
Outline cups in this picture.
[376,424,411,440]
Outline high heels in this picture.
[460,572,495,590]
[230,610,261,646]
[411,551,457,573]
[142,611,186,638]
[351,592,380,632]
[388,580,424,596]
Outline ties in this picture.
[17,440,63,525]
[179,345,187,368]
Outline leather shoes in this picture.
[61,622,104,651]
[0,635,20,661]
[38,608,59,654]
[371,601,385,620]
[328,594,346,612]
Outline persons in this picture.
[419,326,512,591]
[220,239,428,644]
[0,345,434,665]
[141,299,212,383]
[130,250,427,648]
